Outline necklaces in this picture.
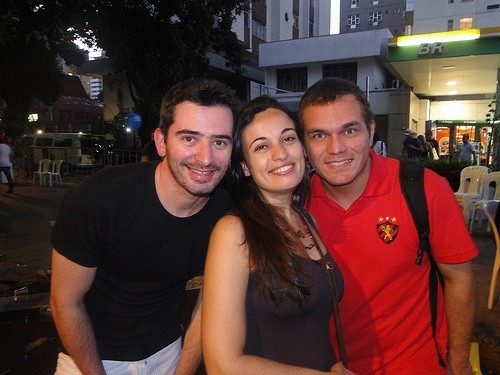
[292,203,315,250]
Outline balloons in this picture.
[126,114,142,133]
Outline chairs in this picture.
[33,159,63,187]
[448,165,500,310]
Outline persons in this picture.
[0,144,13,194]
[49,77,238,375]
[200,94,357,375]
[80,133,161,164]
[452,134,475,164]
[289,78,479,375]
[400,128,441,161]
[370,132,387,159]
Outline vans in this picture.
[11,133,103,178]
